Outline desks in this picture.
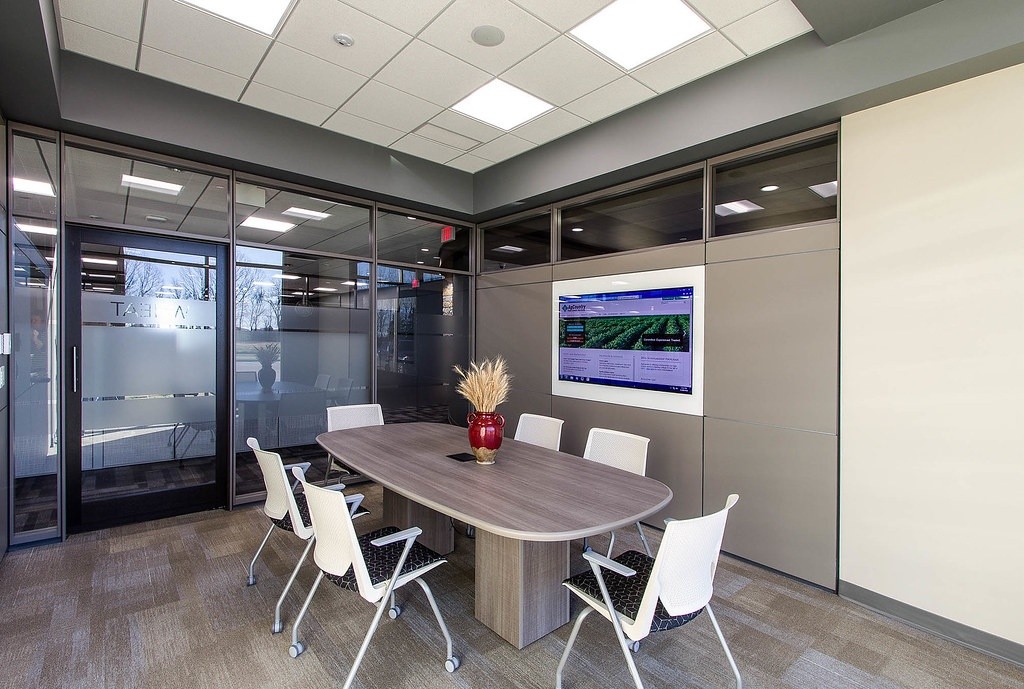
[319,422,673,649]
[211,381,336,449]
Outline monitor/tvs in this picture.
[550,265,706,416]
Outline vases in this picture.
[258,365,276,392]
[467,412,506,465]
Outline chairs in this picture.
[581,427,655,561]
[323,404,385,486]
[235,371,258,382]
[246,437,372,633]
[288,466,461,689]
[315,374,332,390]
[330,377,354,407]
[466,412,564,539]
[265,389,328,446]
[555,493,742,689]
[167,393,216,459]
